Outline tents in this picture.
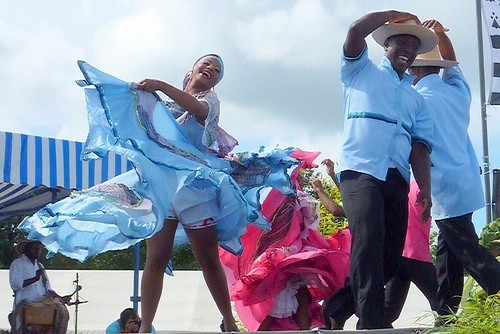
[0,131,141,313]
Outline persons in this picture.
[407,19,500,327]
[308,158,393,329]
[233,159,320,331]
[383,180,438,323]
[106,308,156,334]
[9,240,71,334]
[138,54,241,334]
[322,10,438,330]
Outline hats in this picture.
[408,41,459,66]
[15,237,43,252]
[372,18,437,55]
